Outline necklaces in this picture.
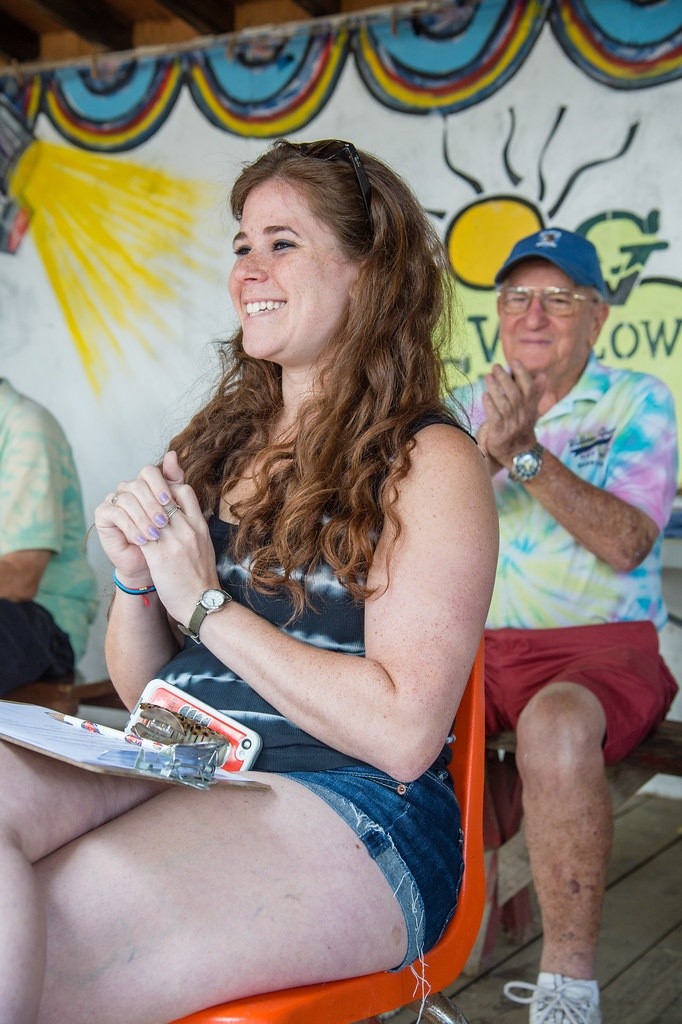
[271,415,298,444]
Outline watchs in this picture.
[177,589,234,646]
[509,444,544,484]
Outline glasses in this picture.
[299,138,371,223]
[500,285,593,317]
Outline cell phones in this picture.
[124,679,262,772]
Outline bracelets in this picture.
[113,570,157,596]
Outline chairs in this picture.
[161,632,484,1024]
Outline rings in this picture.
[111,490,127,507]
[166,505,179,517]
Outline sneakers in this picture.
[503,970,601,1024]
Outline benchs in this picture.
[484,718,681,943]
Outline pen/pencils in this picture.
[45,711,170,752]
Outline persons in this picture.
[442,229,681,1024]
[1,136,500,1024]
[2,376,97,685]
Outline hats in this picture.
[494,228,609,302]
